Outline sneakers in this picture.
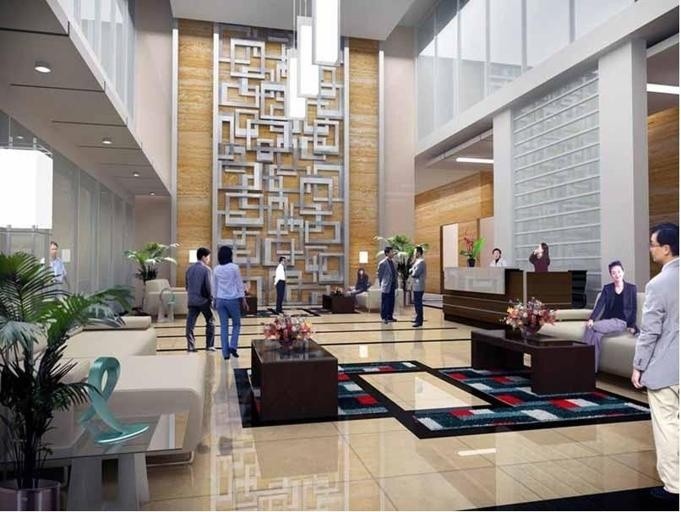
[204,342,217,353]
[187,347,198,353]
[649,486,678,500]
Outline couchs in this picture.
[4,312,215,468]
[537,290,652,379]
[349,277,404,313]
[142,277,189,316]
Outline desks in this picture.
[143,298,189,322]
[1,407,187,512]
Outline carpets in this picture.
[234,352,654,442]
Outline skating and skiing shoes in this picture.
[229,347,244,361]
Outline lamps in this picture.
[358,251,368,264]
[306,0,343,68]
[0,137,54,236]
[187,249,197,263]
[282,0,307,120]
[296,0,321,101]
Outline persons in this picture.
[582,259,640,385]
[40,241,71,291]
[185,247,428,360]
[630,222,678,497]
[528,242,550,271]
[490,247,507,267]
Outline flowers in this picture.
[259,308,315,350]
[495,292,555,339]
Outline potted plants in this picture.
[0,241,139,511]
[374,233,429,305]
[456,235,484,266]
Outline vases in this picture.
[278,334,295,350]
[518,324,541,339]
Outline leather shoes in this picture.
[275,309,285,315]
[383,316,398,326]
[407,319,423,330]
